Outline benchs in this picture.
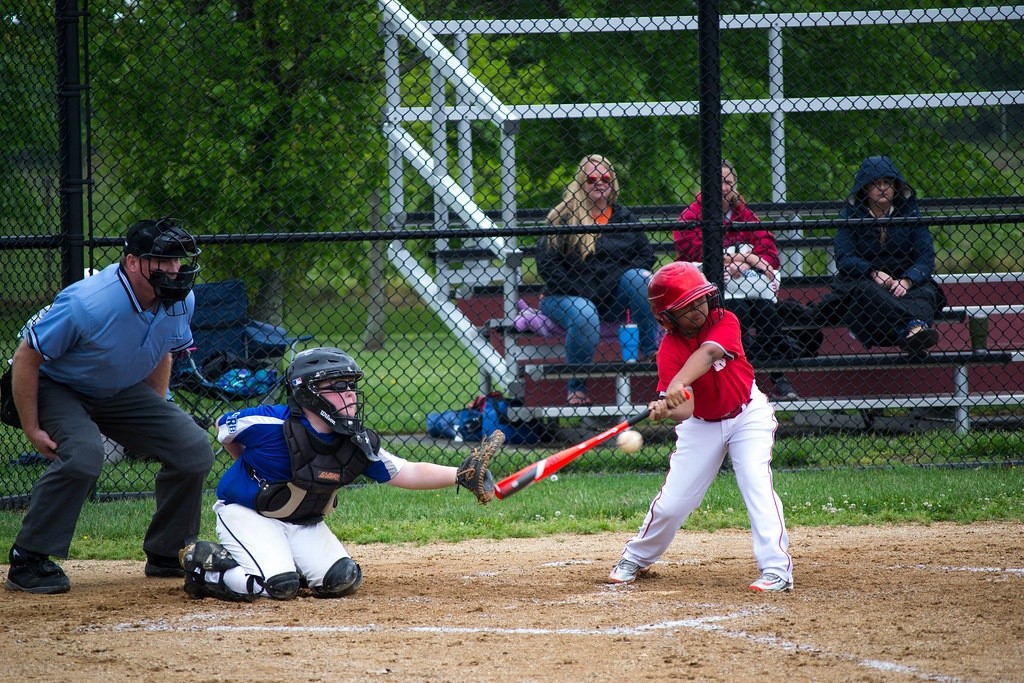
[457,273,1024,436]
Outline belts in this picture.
[693,398,753,422]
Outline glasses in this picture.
[318,379,357,395]
[583,174,613,185]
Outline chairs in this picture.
[166,279,316,458]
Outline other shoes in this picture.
[180,543,208,600]
[896,320,940,353]
[646,350,659,363]
[567,391,593,407]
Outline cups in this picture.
[969,314,989,348]
[618,323,639,364]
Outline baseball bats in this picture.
[493,385,692,499]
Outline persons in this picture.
[537,153,658,405]
[180,347,507,602]
[827,155,948,356]
[672,158,801,400]
[0,217,214,597]
[607,261,796,591]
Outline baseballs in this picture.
[616,430,643,453]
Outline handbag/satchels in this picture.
[425,408,482,442]
[469,390,502,411]
[483,393,549,444]
[694,243,781,304]
[201,349,271,384]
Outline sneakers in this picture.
[144,551,187,578]
[6,543,71,595]
[769,379,800,401]
[608,558,641,584]
[748,572,795,593]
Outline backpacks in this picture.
[755,294,825,358]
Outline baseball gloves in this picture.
[455,429,507,503]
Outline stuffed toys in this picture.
[513,299,553,337]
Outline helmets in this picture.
[124,221,197,259]
[647,260,718,330]
[284,347,366,436]
